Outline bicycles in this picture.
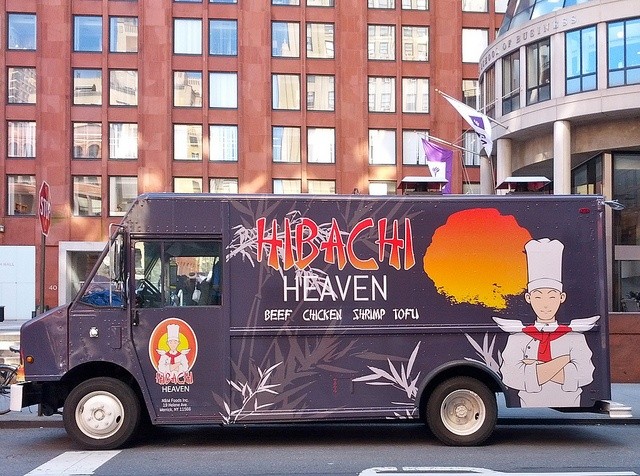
[0,345,37,413]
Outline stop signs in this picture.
[38,181,51,237]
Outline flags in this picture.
[417,136,454,194]
[441,94,494,158]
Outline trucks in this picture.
[17,176,632,450]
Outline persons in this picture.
[157,323,188,378]
